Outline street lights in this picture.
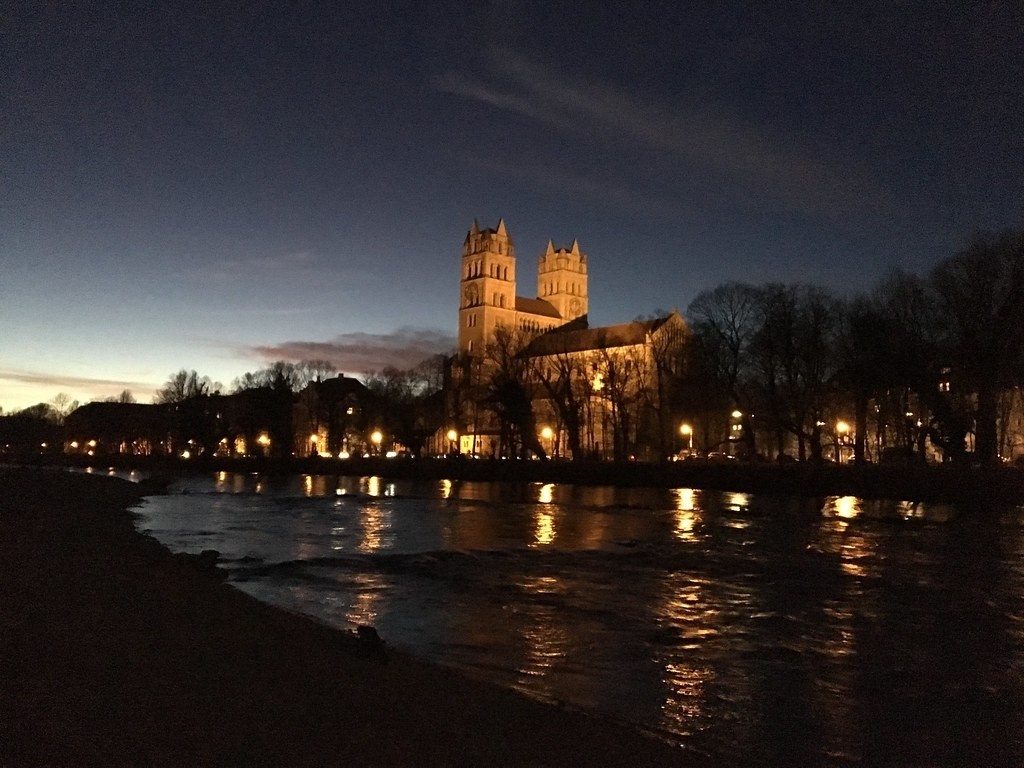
[749,411,756,422]
[38,441,49,460]
[591,372,607,457]
[259,435,270,456]
[160,439,163,460]
[214,412,222,429]
[539,422,557,458]
[814,419,831,460]
[833,416,857,465]
[678,424,695,467]
[185,438,193,458]
[903,407,916,425]
[310,432,321,456]
[344,403,353,430]
[444,427,463,459]
[370,426,386,461]
[70,440,77,455]
[730,402,745,463]
[86,436,97,460]
[3,439,10,457]
[122,440,127,461]
[221,435,226,455]
